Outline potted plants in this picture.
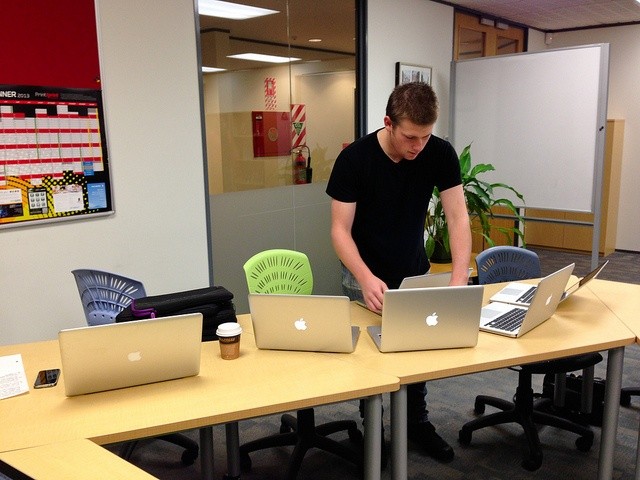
[425,142,527,263]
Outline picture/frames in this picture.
[396,61,433,89]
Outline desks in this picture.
[0,439,159,479]
[0,314,400,479]
[352,275,636,480]
[427,250,496,284]
[583,277,640,350]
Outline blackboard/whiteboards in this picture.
[452,41,606,224]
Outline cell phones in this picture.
[33,368,60,389]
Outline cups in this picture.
[215,320,243,360]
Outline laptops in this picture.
[58,312,204,397]
[489,259,610,307]
[248,294,360,353]
[355,268,473,317]
[367,284,484,353]
[479,262,576,338]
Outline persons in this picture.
[325,81,473,472]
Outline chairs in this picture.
[71,268,199,465]
[457,247,594,472]
[239,249,364,480]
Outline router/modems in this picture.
[542,372,606,428]
